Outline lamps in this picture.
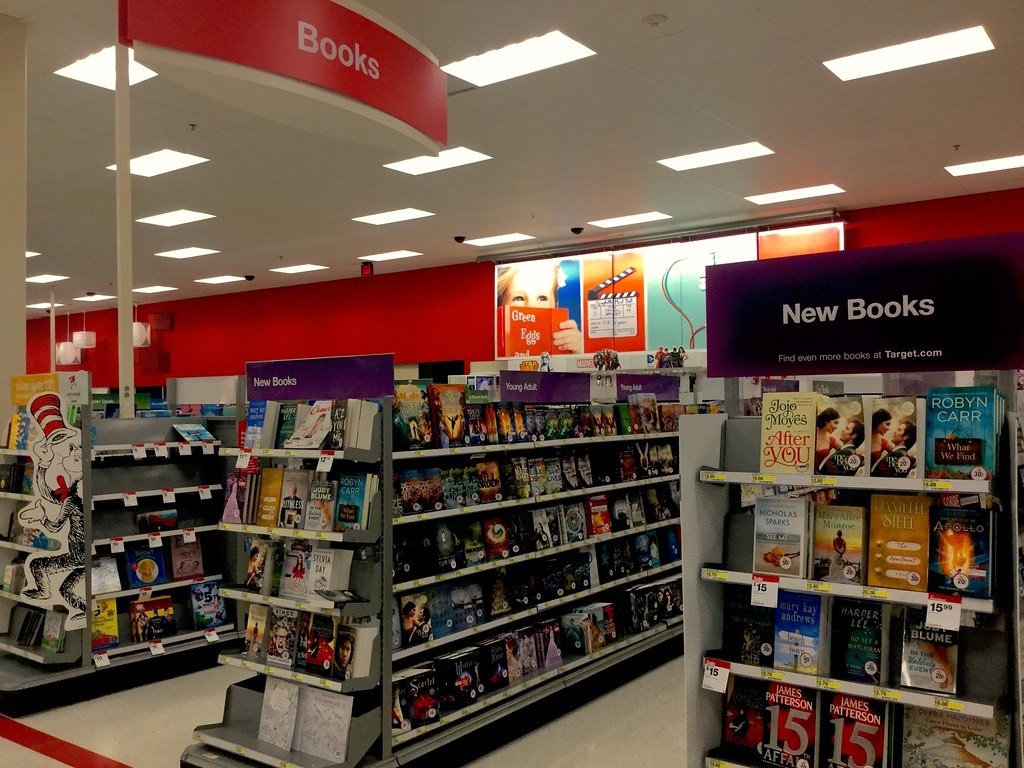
[133,302,151,348]
[55,311,81,365]
[73,310,96,348]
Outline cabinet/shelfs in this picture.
[679,376,1024,768]
[0,405,250,720]
[180,390,686,768]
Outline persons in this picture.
[248,545,259,585]
[253,622,259,644]
[279,484,301,529]
[302,414,326,439]
[402,602,431,645]
[823,530,860,585]
[505,614,604,680]
[657,588,680,618]
[497,260,581,355]
[333,631,355,680]
[816,407,918,478]
[268,628,290,658]
[292,553,305,579]
